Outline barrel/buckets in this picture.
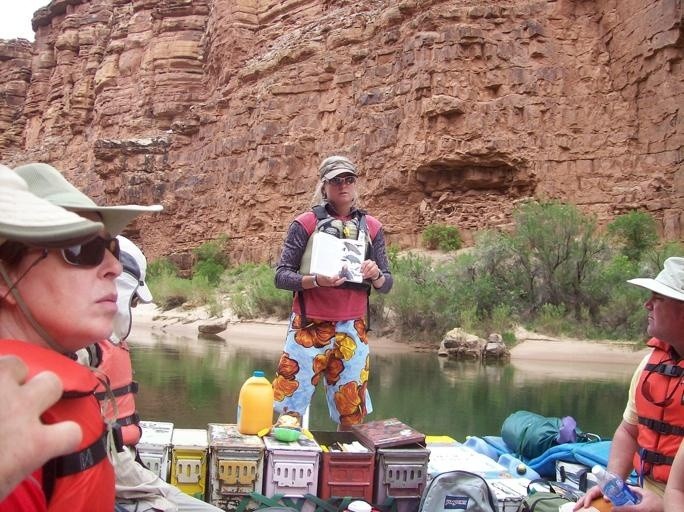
[236,371,273,435]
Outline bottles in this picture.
[591,465,640,506]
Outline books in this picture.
[310,231,367,284]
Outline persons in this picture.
[662,436,683,512]
[1,164,164,511]
[1,164,103,504]
[76,235,228,512]
[571,257,684,512]
[272,155,394,432]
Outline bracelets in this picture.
[375,269,384,282]
[312,276,317,287]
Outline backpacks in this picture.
[417,470,499,512]
[515,478,573,512]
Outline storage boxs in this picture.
[137,420,433,512]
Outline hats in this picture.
[319,155,360,183]
[11,163,164,239]
[0,163,105,242]
[626,256,684,302]
[106,234,153,346]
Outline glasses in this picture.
[324,176,356,186]
[59,235,120,268]
